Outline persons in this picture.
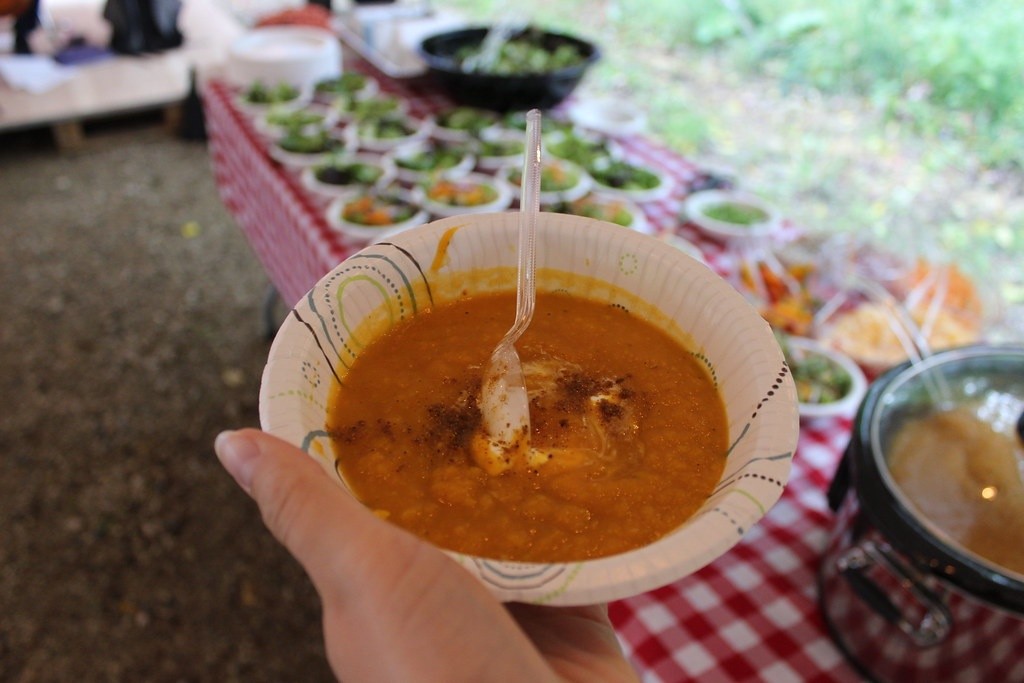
[210,427,641,683]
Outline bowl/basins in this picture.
[419,25,599,110]
[258,210,800,607]
[229,76,872,414]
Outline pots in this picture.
[818,343,1024,683]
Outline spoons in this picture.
[477,108,543,442]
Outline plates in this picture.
[229,26,342,89]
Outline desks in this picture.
[0,0,1024,683]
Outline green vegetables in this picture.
[242,32,767,238]
